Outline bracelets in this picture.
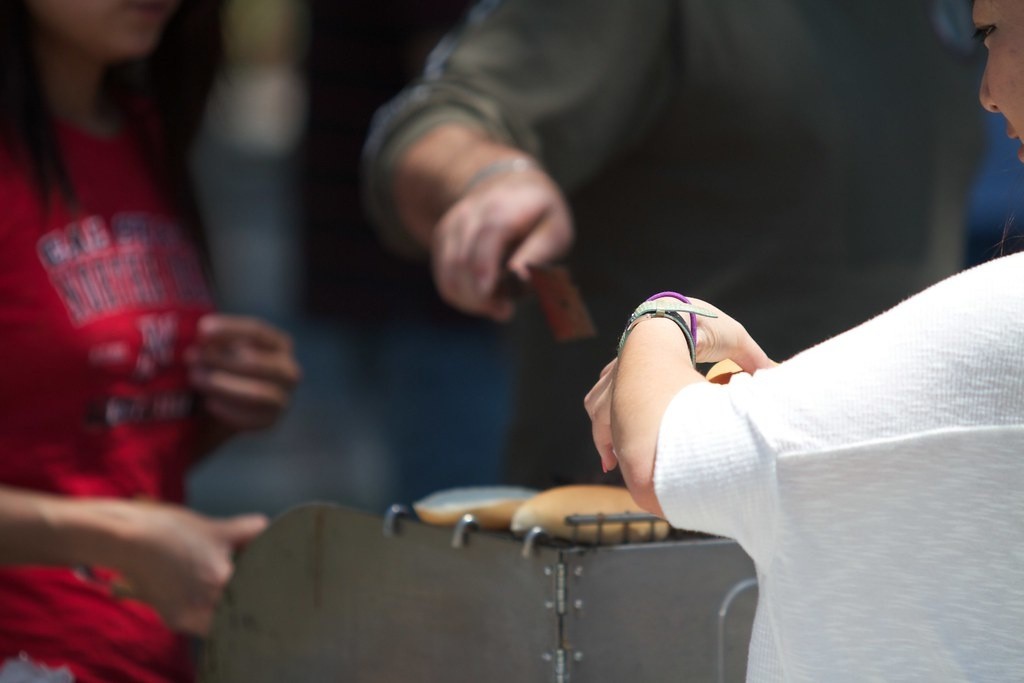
[616,290,718,369]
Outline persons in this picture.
[372,1,989,494]
[0,1,297,683]
[584,1,1024,683]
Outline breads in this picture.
[508,484,669,546]
[414,487,542,529]
